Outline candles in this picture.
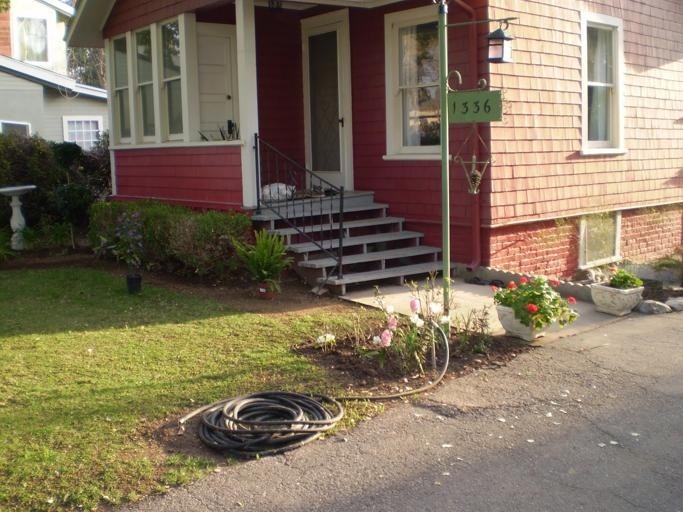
[472,150,476,171]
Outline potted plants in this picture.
[116,211,143,294]
[235,232,295,299]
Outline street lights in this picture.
[436,1,522,315]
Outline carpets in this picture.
[294,189,325,198]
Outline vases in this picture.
[590,282,643,317]
[497,306,548,339]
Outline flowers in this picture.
[495,277,577,329]
[608,267,643,290]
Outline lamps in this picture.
[487,21,513,62]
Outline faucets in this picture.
[428,315,441,331]
